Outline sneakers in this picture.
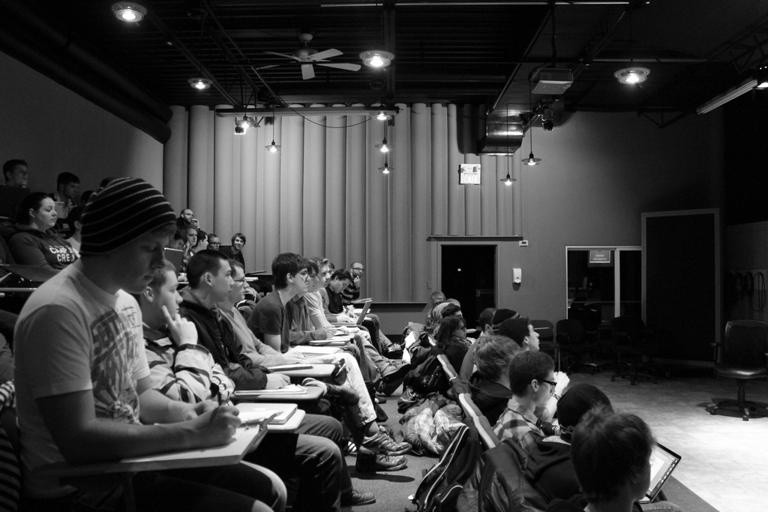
[359,432,412,455]
[372,368,402,421]
[341,489,377,506]
[355,453,408,471]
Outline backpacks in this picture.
[410,425,482,512]
[396,356,444,413]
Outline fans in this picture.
[255,33,361,82]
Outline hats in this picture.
[492,309,522,335]
[79,176,178,255]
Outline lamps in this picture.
[693,75,758,116]
[107,0,282,155]
[610,0,651,87]
[500,102,515,186]
[518,76,541,169]
[357,1,397,176]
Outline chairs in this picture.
[0,214,371,512]
[407,315,672,512]
[701,318,767,420]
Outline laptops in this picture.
[636,441,681,502]
[330,300,373,327]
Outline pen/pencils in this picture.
[268,366,313,372]
[217,392,223,406]
